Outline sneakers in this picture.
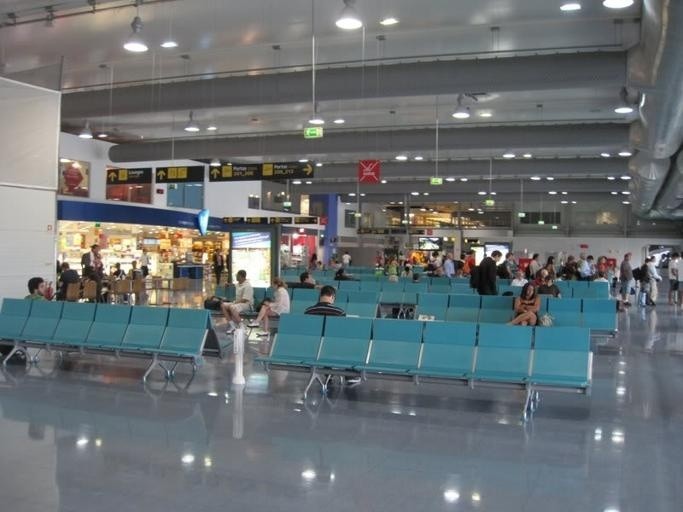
[226,328,234,335]
[256,330,269,336]
[247,321,260,328]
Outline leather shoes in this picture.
[624,302,631,306]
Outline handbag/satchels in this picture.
[204,295,223,311]
[538,314,556,327]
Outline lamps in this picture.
[78,119,93,140]
[452,95,470,119]
[309,96,325,125]
[336,1,362,31]
[615,87,633,113]
[602,0,633,10]
[184,110,200,132]
[124,2,148,54]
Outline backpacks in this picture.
[470,266,482,289]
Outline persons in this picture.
[23,245,151,302]
[201,235,352,335]
[620,253,683,305]
[476,250,502,295]
[505,252,614,297]
[509,283,539,326]
[376,249,475,284]
